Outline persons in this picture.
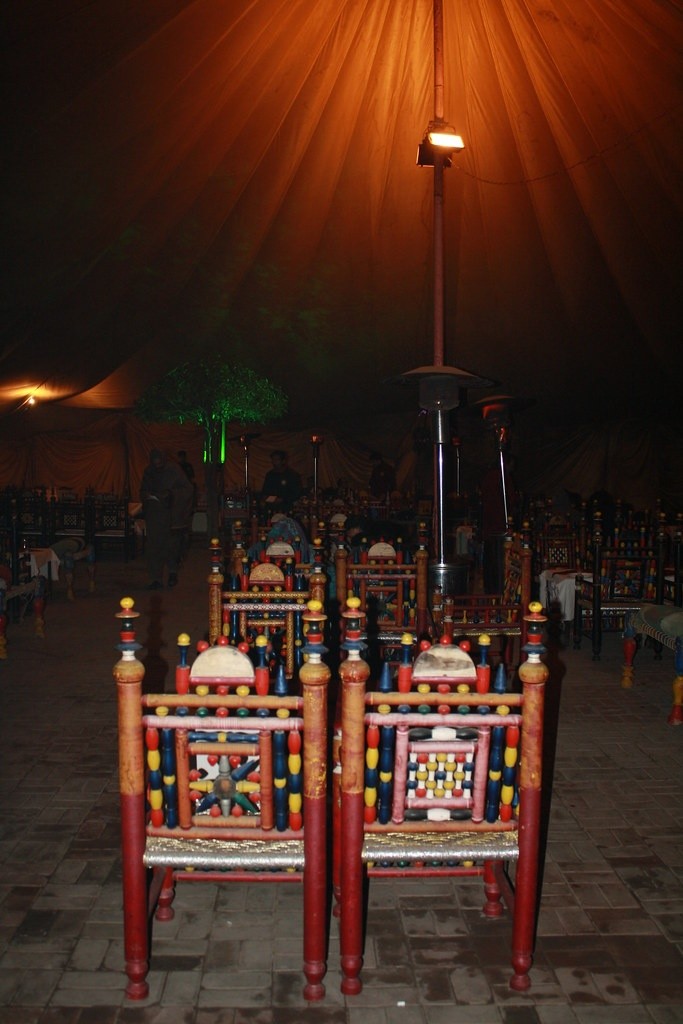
[241,450,410,604]
[177,450,195,566]
[477,453,521,594]
[139,446,194,590]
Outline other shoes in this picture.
[144,580,163,590]
[169,572,176,586]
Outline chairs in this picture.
[0,485,683,1004]
